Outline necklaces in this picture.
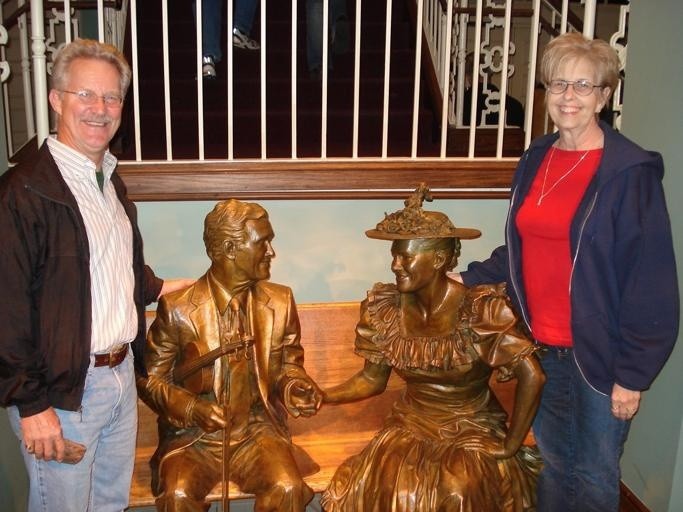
[536,143,592,206]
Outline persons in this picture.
[0,35,202,512]
[135,199,327,512]
[442,31,682,512]
[312,180,548,512]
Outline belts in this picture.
[95,343,128,370]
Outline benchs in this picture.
[129,299,537,506]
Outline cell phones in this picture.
[54,436,86,466]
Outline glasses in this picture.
[545,80,607,97]
[58,87,126,109]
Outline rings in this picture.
[26,444,34,453]
